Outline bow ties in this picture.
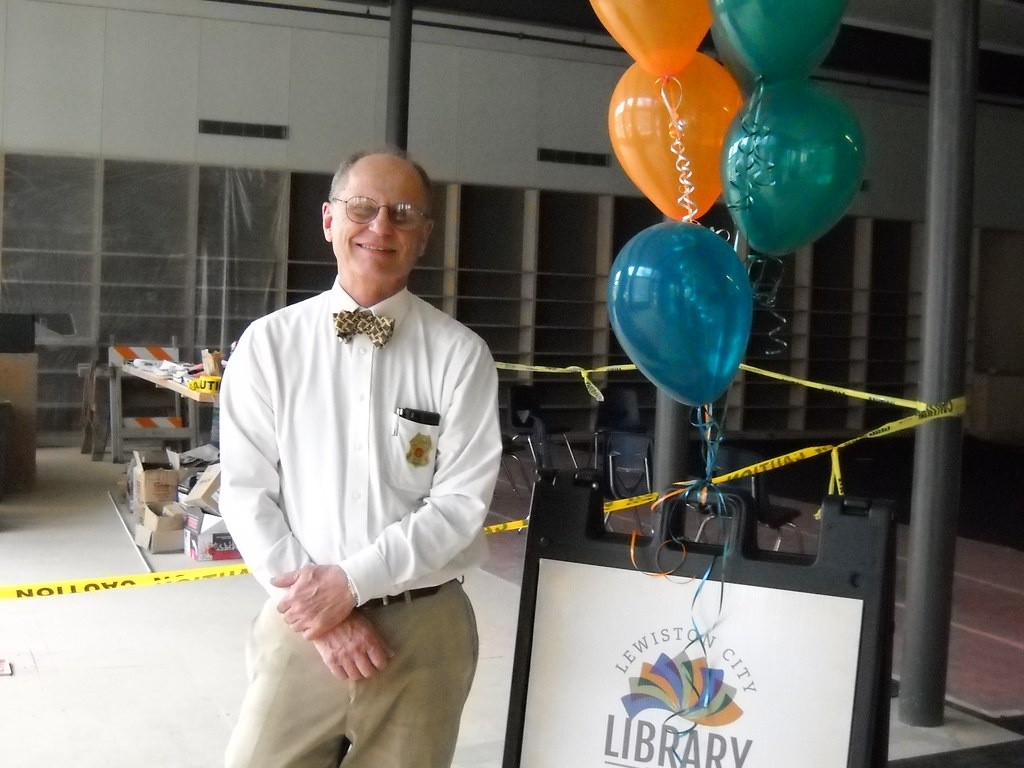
[333,309,395,349]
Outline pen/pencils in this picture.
[393,408,403,435]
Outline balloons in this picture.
[590,0,863,409]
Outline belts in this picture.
[355,585,441,610]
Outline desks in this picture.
[108,363,219,465]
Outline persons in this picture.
[218,147,503,768]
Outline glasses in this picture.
[332,196,430,231]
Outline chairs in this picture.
[497,383,804,556]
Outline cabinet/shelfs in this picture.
[0,353,40,489]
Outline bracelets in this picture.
[347,576,358,608]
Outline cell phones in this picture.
[402,407,440,426]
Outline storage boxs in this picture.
[0,312,36,353]
[131,450,242,560]
